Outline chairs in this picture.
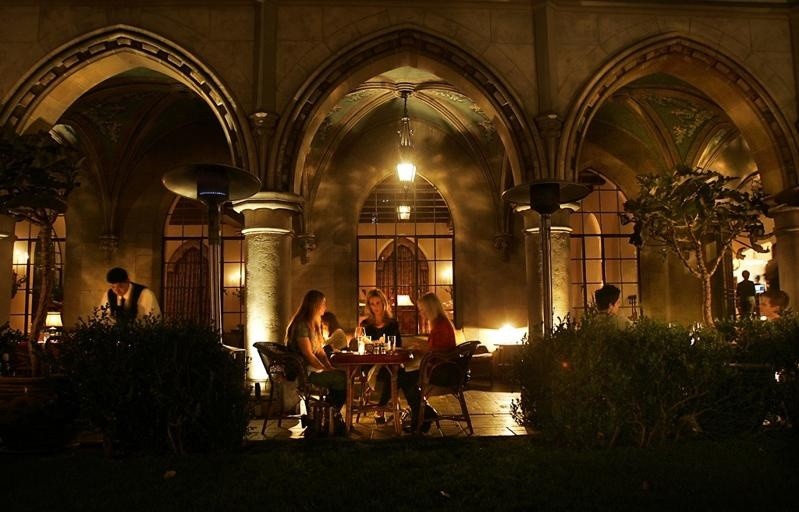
[253,337,481,438]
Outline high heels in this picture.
[373,406,440,433]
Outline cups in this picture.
[358,336,371,354]
[387,336,396,353]
[355,326,367,341]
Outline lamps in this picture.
[46,312,63,335]
[396,97,418,221]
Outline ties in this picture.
[120,298,126,306]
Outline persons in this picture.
[758,289,792,323]
[580,285,636,339]
[283,290,354,438]
[319,312,348,351]
[399,291,458,435]
[355,287,404,426]
[734,269,757,323]
[94,266,161,329]
